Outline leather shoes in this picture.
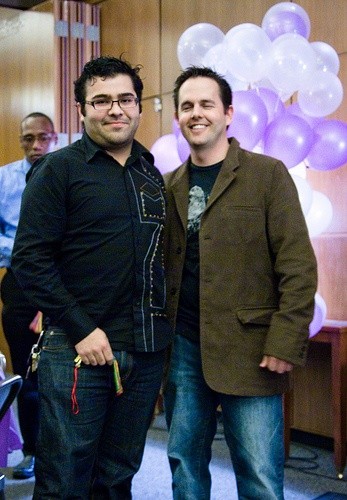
[12,455,37,478]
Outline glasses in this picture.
[85,98,140,111]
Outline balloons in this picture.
[307,292,326,338]
[150,1,346,238]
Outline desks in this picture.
[281,319,347,479]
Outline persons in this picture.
[154,67,317,500]
[0,111,67,478]
[8,54,166,500]
